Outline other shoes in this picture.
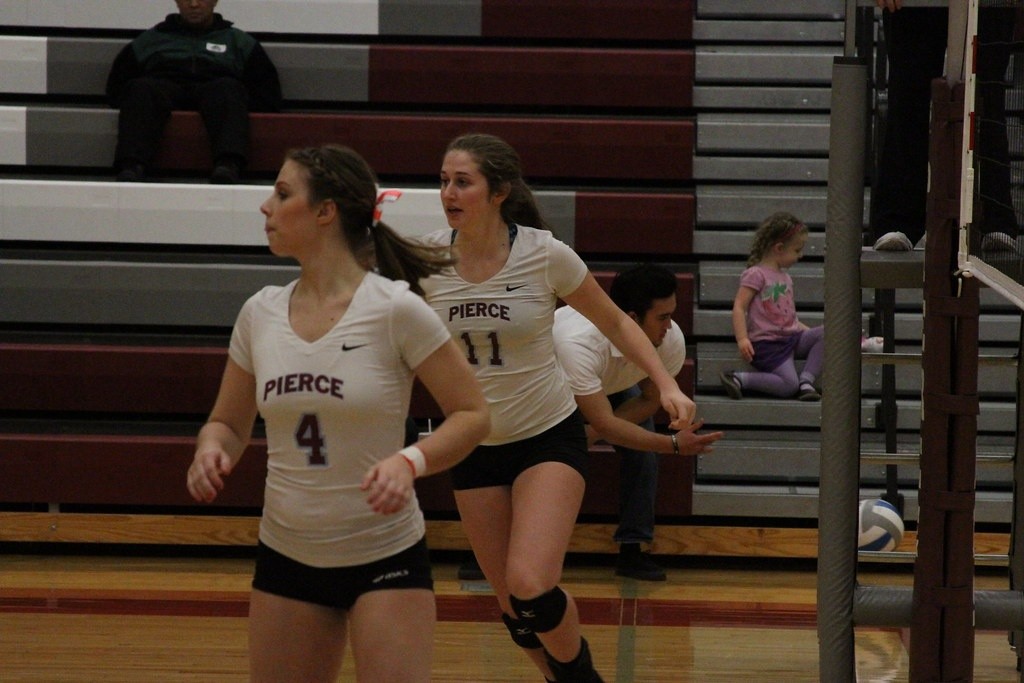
[211,163,249,185]
[119,166,147,181]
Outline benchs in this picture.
[0,2,1024,531]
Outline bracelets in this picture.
[398,445,426,478]
[672,434,680,456]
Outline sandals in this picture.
[797,381,822,401]
[721,371,743,400]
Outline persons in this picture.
[717,209,825,403]
[186,145,491,681]
[399,135,699,683]
[872,1,1022,258]
[539,262,692,580]
[101,0,285,182]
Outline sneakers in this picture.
[459,553,485,580]
[872,230,913,252]
[980,232,1020,254]
[615,551,667,581]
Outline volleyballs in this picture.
[858,499,905,552]
